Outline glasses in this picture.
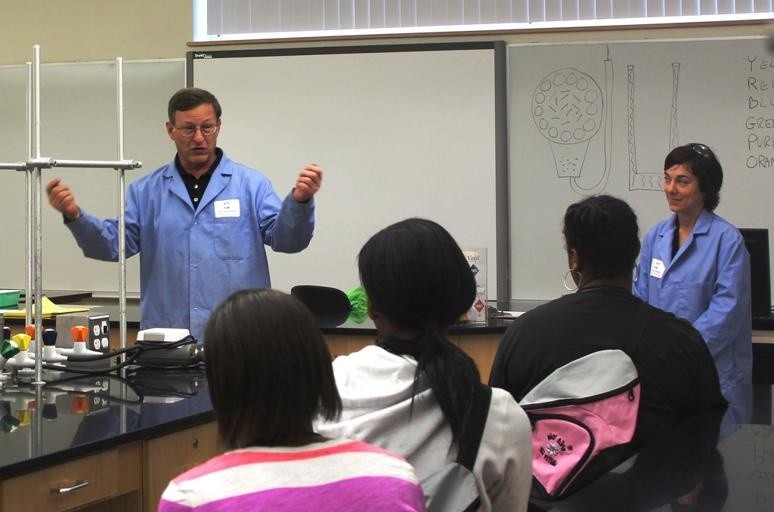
[172,122,218,136]
[687,143,715,161]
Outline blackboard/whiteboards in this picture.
[0,59,186,299]
[506,36,774,313]
[185,41,507,329]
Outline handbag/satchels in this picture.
[522,349,642,501]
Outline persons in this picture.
[45,86,323,344]
[159,288,428,512]
[313,215,534,512]
[632,142,755,423]
[487,196,731,512]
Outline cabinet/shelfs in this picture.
[0,440,144,512]
[144,421,225,512]
